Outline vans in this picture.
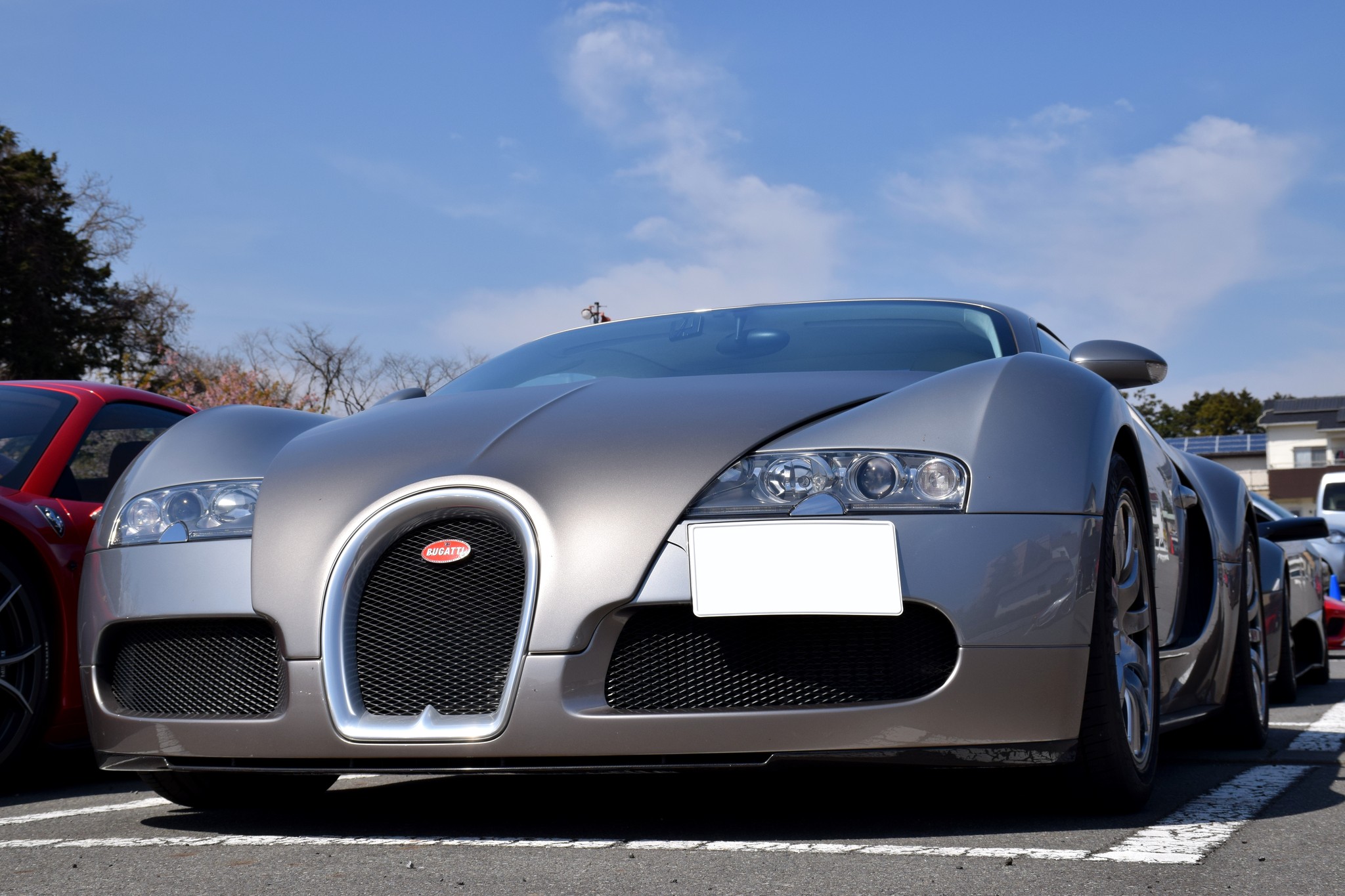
[1314,472,1345,538]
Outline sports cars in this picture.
[0,294,1331,815]
